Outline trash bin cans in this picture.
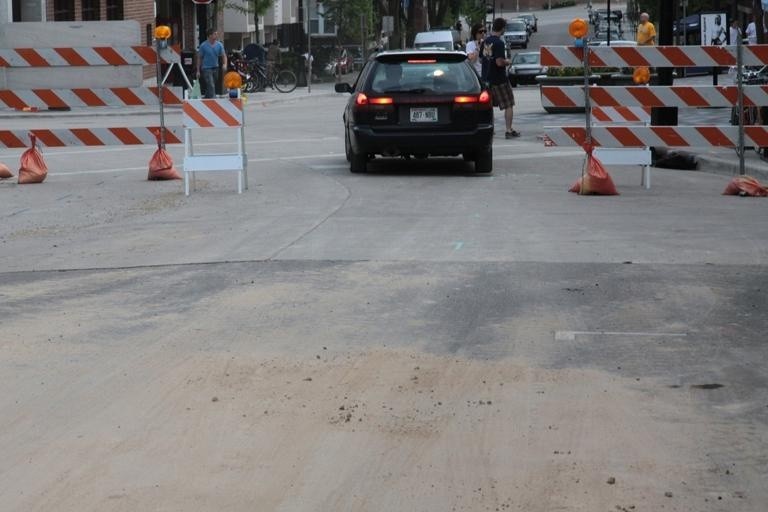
[173,51,194,99]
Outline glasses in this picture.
[478,29,487,34]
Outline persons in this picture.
[710,16,729,46]
[264,38,281,89]
[479,17,521,139]
[745,20,768,44]
[466,23,485,76]
[634,12,657,85]
[583,0,596,25]
[300,47,314,87]
[196,28,229,98]
[728,17,743,46]
[325,33,390,74]
[455,21,464,31]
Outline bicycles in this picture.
[226,48,299,93]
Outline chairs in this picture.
[379,74,458,90]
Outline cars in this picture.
[589,8,637,47]
[730,65,767,163]
[334,14,549,174]
[673,14,729,78]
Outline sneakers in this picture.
[506,128,519,137]
